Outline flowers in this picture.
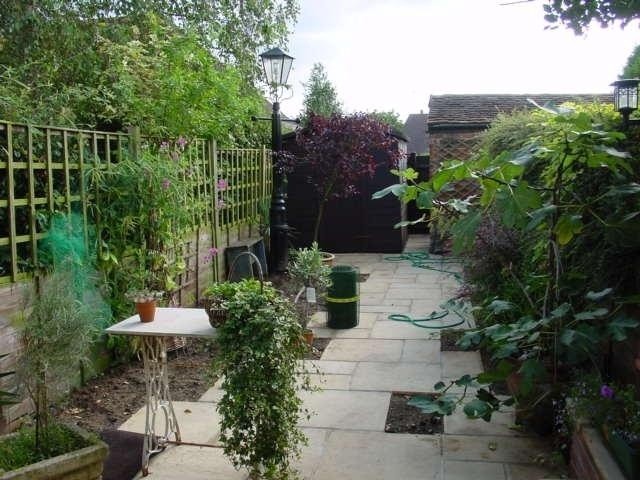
[548,364,640,456]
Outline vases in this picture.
[600,423,639,480]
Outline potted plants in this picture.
[264,106,413,278]
[2,253,113,480]
[198,277,325,480]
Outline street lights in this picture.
[255,45,295,282]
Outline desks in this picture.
[102,305,224,477]
